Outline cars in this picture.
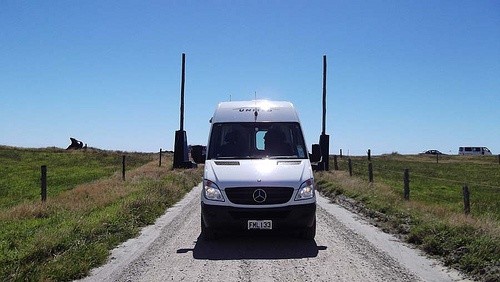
[419,149,447,156]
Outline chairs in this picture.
[265,130,292,156]
[220,132,247,156]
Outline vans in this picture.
[190,100,322,242]
[459,146,493,156]
